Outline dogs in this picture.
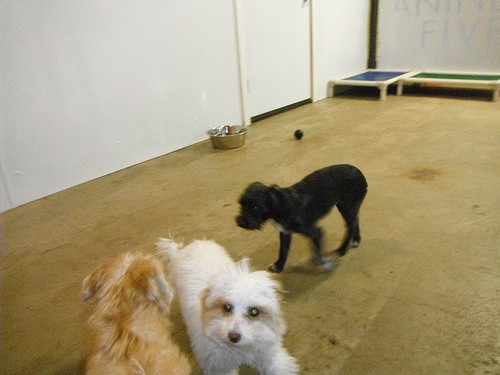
[154,237,299,375]
[238,164,368,276]
[79,250,191,375]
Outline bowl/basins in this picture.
[207,127,246,149]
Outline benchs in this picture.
[328,67,413,101]
[395,70,500,102]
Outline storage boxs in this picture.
[208,124,249,150]
